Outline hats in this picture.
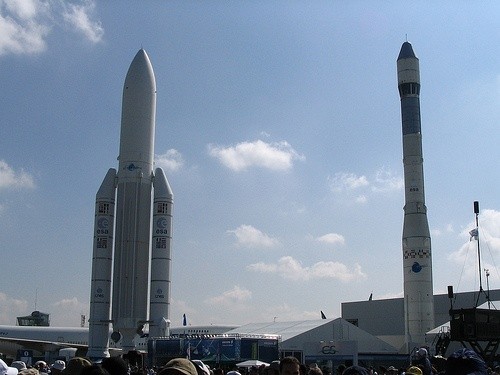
[158,357,197,375]
[0,359,9,375]
[446,349,488,375]
[11,361,27,369]
[342,366,369,375]
[66,358,93,373]
[416,348,429,357]
[192,360,211,375]
[6,367,19,375]
[18,368,38,374]
[52,360,64,371]
[404,366,423,374]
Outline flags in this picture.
[469,228,478,241]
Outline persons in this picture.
[1,348,500,375]
